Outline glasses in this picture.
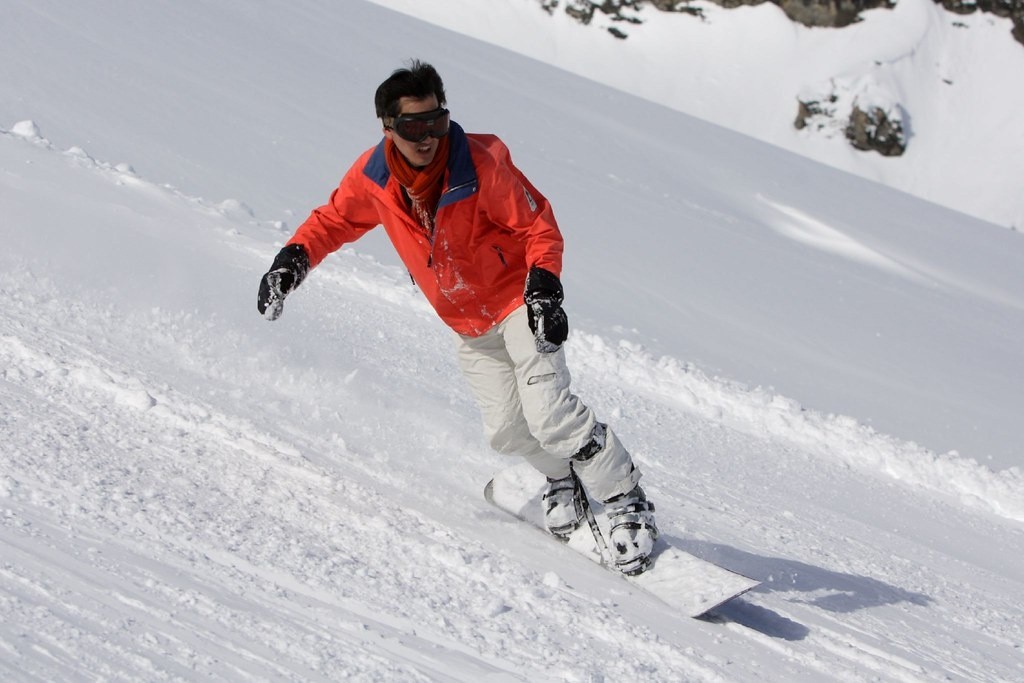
[393,106,449,142]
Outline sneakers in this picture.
[543,476,587,535]
[602,483,658,576]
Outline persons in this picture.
[258,60,661,577]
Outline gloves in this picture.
[523,263,569,354]
[257,243,308,321]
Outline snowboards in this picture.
[483,462,765,620]
